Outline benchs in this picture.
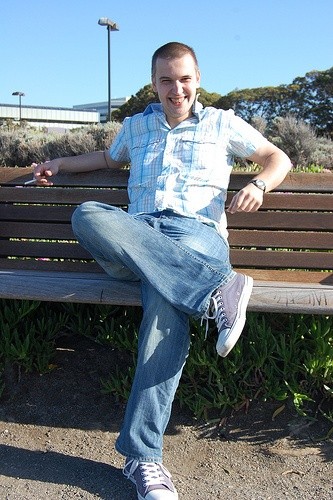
[0,164,333,315]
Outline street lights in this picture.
[12,91,26,122]
[97,17,121,124]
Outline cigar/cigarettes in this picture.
[24,175,46,185]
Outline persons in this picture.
[32,42,293,500]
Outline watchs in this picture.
[247,179,268,193]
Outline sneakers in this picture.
[210,272,253,357]
[123,457,178,500]
